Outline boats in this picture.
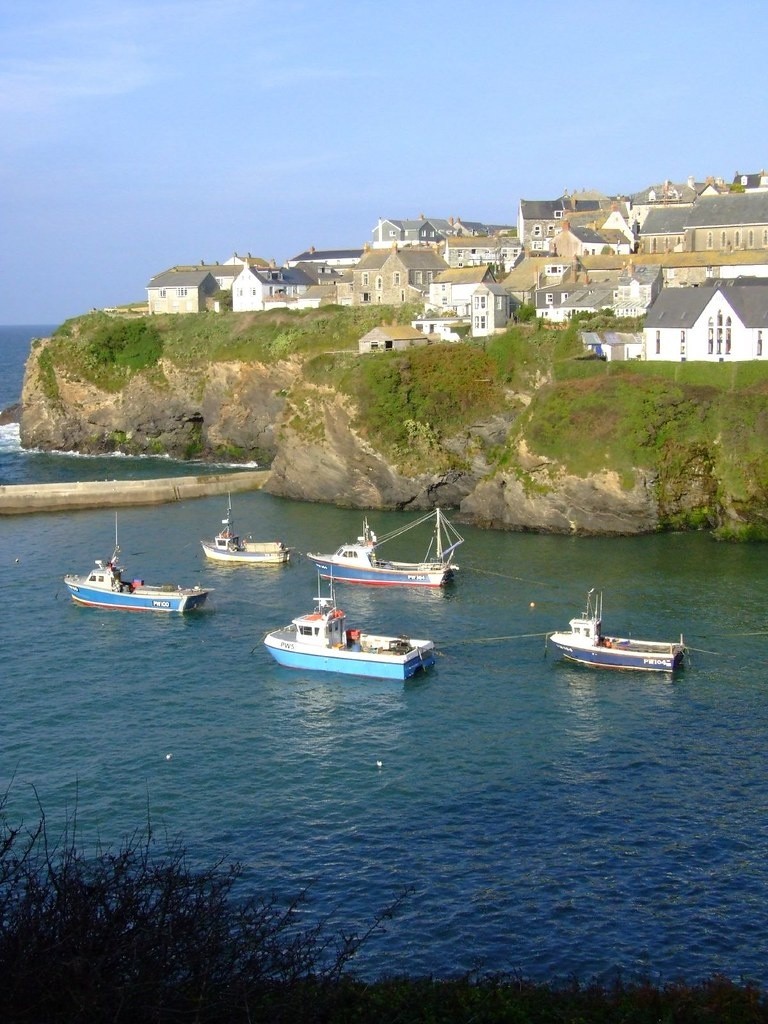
[257,564,438,681]
[306,506,466,587]
[549,587,687,674]
[200,489,296,564]
[63,512,216,613]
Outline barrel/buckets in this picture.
[346,629,361,652]
